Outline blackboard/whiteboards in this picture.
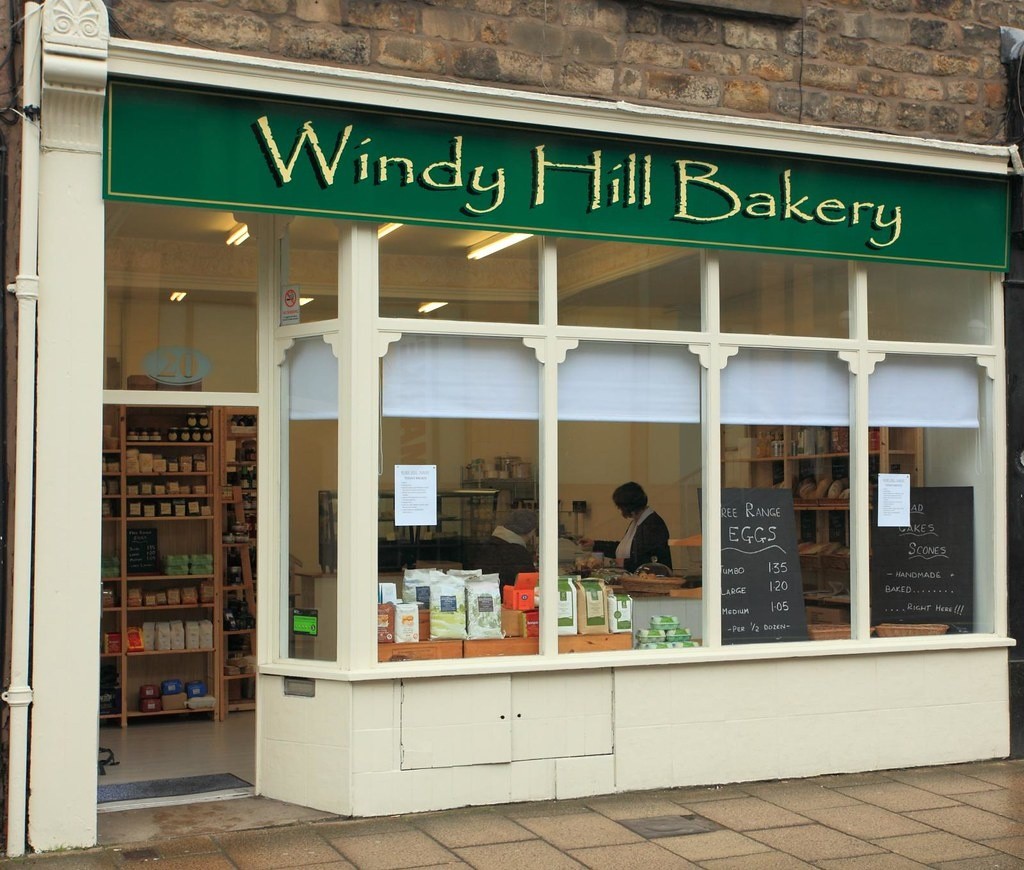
[697,486,810,645]
[870,486,975,637]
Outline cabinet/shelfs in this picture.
[99,407,220,728]
[222,406,259,715]
[749,427,924,601]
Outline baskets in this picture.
[875,624,949,637]
[794,498,850,506]
[797,539,850,571]
[620,577,687,593]
[807,624,874,640]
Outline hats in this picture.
[504,510,537,534]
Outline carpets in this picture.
[97,771,253,805]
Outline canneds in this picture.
[222,446,257,510]
[770,440,798,456]
[127,412,212,443]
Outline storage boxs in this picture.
[127,449,206,473]
[139,680,216,712]
[127,482,206,495]
[162,554,213,575]
[129,502,211,518]
[103,631,121,653]
[503,573,538,610]
[101,557,120,578]
[127,583,213,607]
[226,440,236,462]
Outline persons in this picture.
[578,481,673,577]
[461,509,537,604]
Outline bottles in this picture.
[467,454,532,482]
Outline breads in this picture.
[102,580,214,609]
[798,542,849,557]
[794,475,876,500]
[575,557,601,569]
